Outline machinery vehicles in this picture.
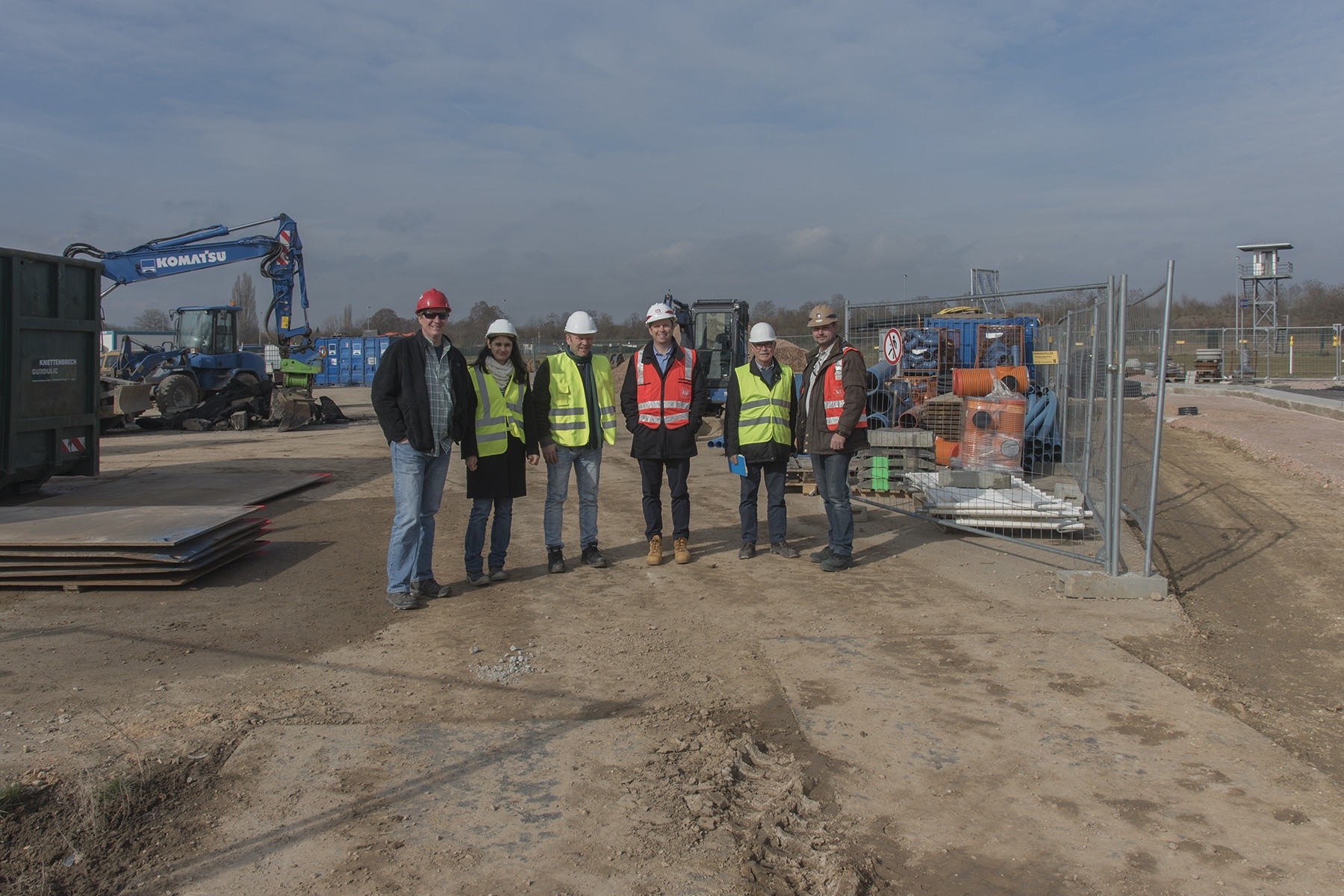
[62,212,324,433]
[661,294,748,407]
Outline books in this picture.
[727,455,748,477]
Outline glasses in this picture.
[422,309,448,320]
[754,341,773,350]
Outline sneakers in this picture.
[810,546,835,563]
[547,546,566,573]
[466,571,490,586]
[646,535,662,565]
[580,541,606,567]
[674,537,691,563]
[821,553,854,571]
[739,542,756,559]
[386,591,419,610]
[488,565,508,580]
[410,577,452,598]
[770,540,798,558]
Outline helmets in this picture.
[645,302,677,327]
[564,310,597,335]
[807,304,840,327]
[485,319,518,339]
[749,322,777,343]
[414,288,452,315]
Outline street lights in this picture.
[367,305,370,331]
[902,275,909,328]
[503,300,507,319]
[259,312,262,347]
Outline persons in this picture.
[371,289,471,608]
[531,311,616,574]
[795,304,870,572]
[724,322,801,560]
[620,303,706,564]
[461,319,540,586]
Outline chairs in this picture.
[716,334,730,349]
[202,324,227,354]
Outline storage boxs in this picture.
[315,337,402,387]
[0,247,104,493]
